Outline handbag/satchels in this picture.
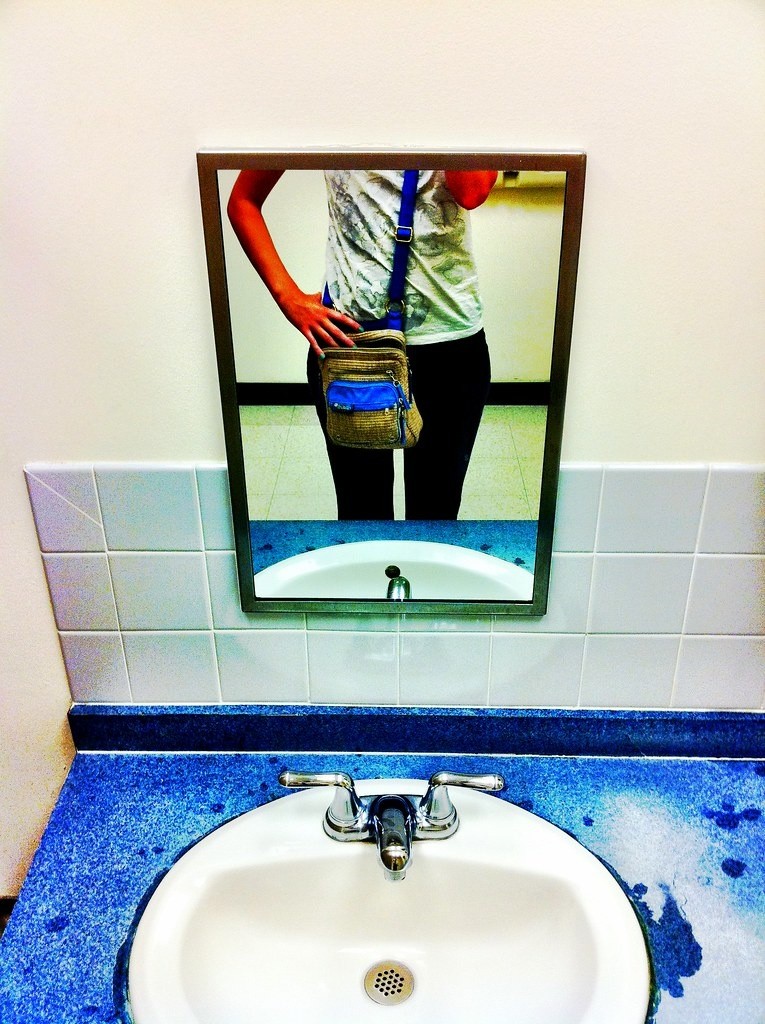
[319,314,424,452]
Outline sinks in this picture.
[253,538,534,600]
[126,776,653,1023]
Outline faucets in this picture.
[369,793,417,885]
[383,565,412,599]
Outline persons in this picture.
[228,168,497,520]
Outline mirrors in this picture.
[193,150,589,617]
